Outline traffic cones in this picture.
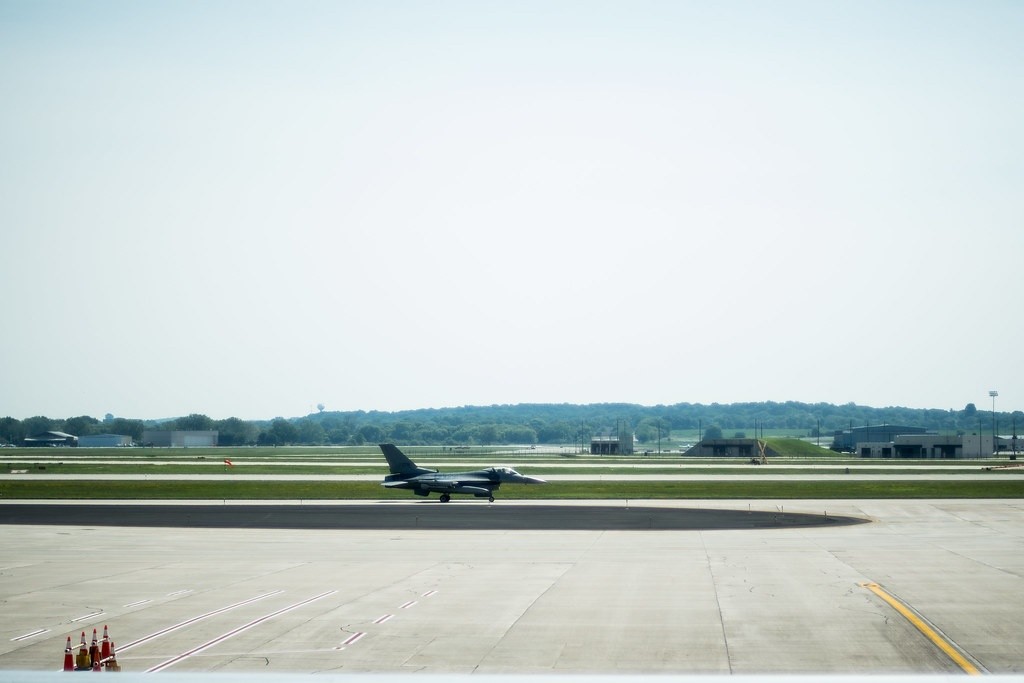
[63,624,121,673]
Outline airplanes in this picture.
[379,443,548,503]
